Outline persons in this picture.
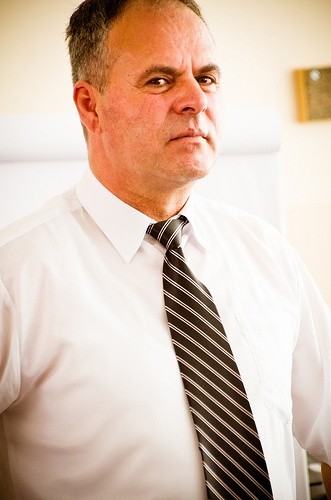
[0,1,331,499]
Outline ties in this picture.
[147,218,274,499]
